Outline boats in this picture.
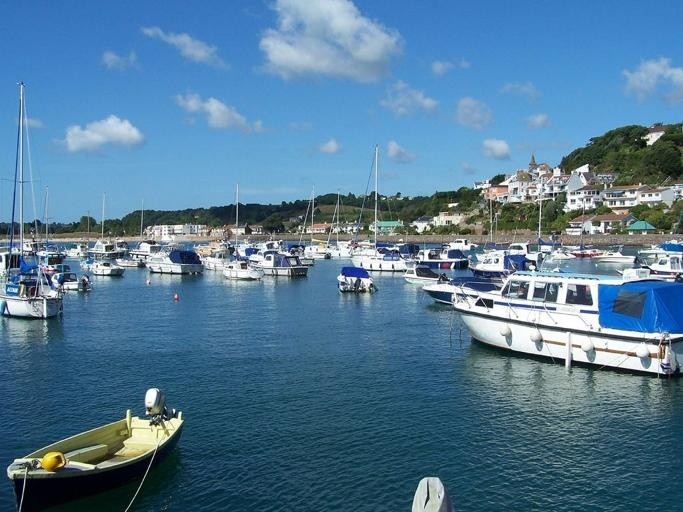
[5,387,186,512]
